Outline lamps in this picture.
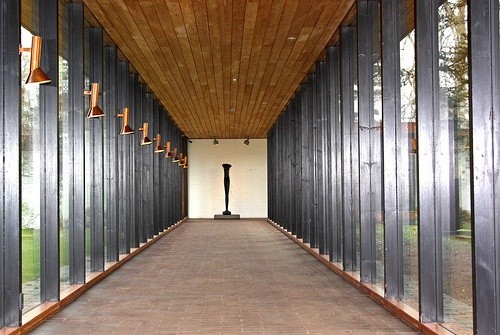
[83,82,105,119]
[172,145,180,163]
[138,122,152,146]
[178,152,184,166]
[117,107,135,135]
[244,137,250,145]
[163,141,172,158]
[18,34,50,84]
[182,157,189,169]
[152,133,164,153]
[213,138,218,145]
